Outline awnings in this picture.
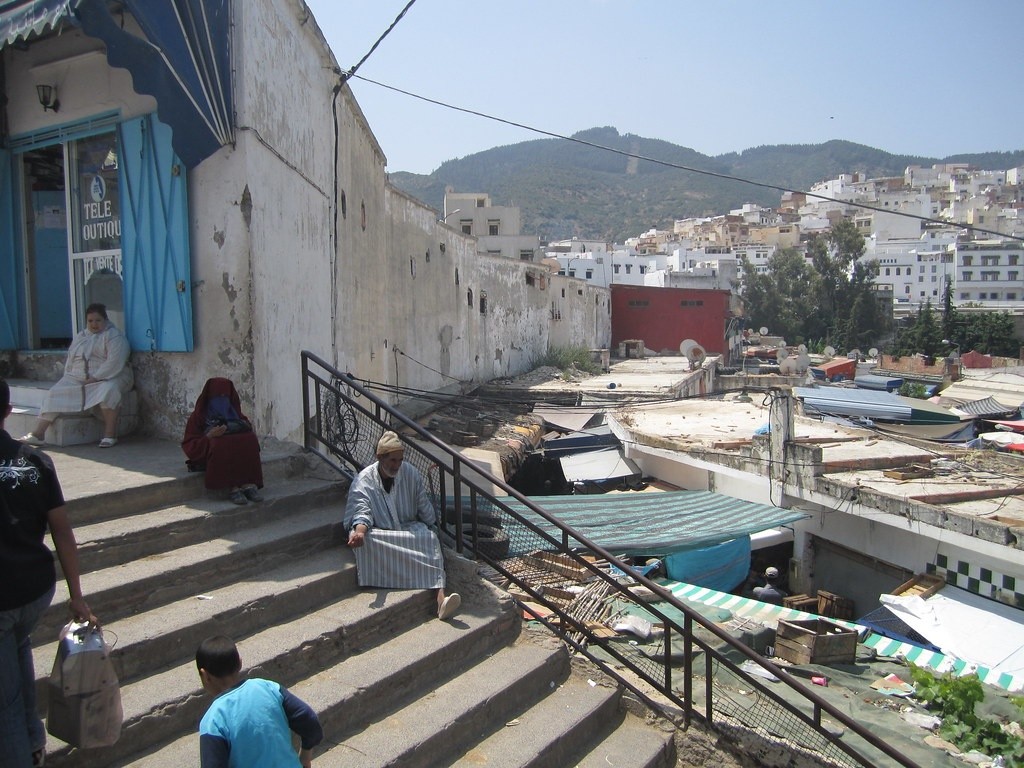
[0,0,236,171]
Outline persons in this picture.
[196,634,323,768]
[181,377,264,506]
[14,303,135,448]
[0,378,104,768]
[343,430,462,621]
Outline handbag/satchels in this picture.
[44,618,123,749]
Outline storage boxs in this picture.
[46,680,119,747]
[784,590,855,618]
[773,617,858,667]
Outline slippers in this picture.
[99,437,118,448]
[13,433,49,446]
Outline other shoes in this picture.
[33,748,46,767]
[242,487,265,502]
[439,592,462,621]
[229,488,248,504]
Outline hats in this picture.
[377,431,404,455]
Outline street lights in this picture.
[942,340,960,379]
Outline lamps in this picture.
[35,85,59,112]
